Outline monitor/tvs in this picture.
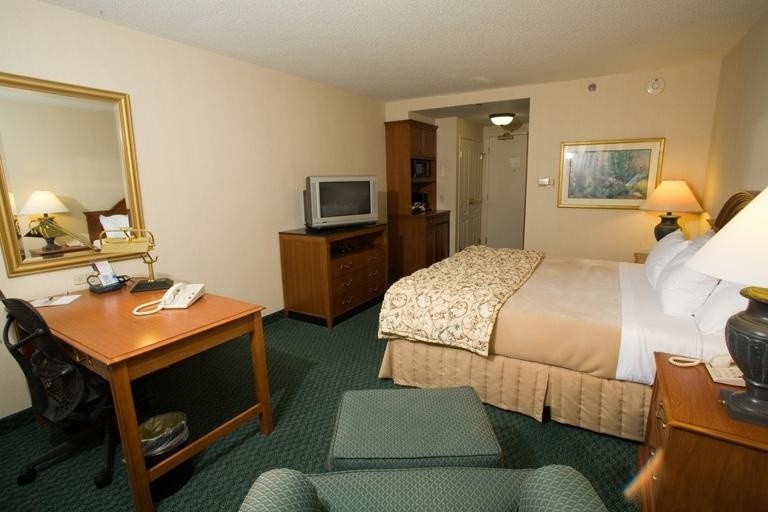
[303,174,379,233]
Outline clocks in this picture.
[646,76,664,95]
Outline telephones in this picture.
[154,282,206,310]
[699,353,746,387]
[65,239,86,246]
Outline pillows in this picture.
[99,215,131,239]
[655,233,719,318]
[644,229,691,287]
[693,280,758,334]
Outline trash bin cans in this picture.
[136,411,194,503]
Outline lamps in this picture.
[18,191,71,250]
[488,113,515,126]
[639,181,703,242]
[685,185,768,427]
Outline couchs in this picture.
[238,465,607,512]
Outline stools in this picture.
[327,385,504,473]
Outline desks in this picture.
[13,276,274,512]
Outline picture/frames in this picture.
[557,137,665,210]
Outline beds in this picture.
[82,198,129,243]
[376,191,761,444]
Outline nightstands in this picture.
[29,245,93,258]
[633,253,648,263]
[636,351,768,511]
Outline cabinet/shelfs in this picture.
[279,223,389,328]
[383,120,450,276]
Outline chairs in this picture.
[0,292,156,488]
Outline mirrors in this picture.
[0,71,150,278]
